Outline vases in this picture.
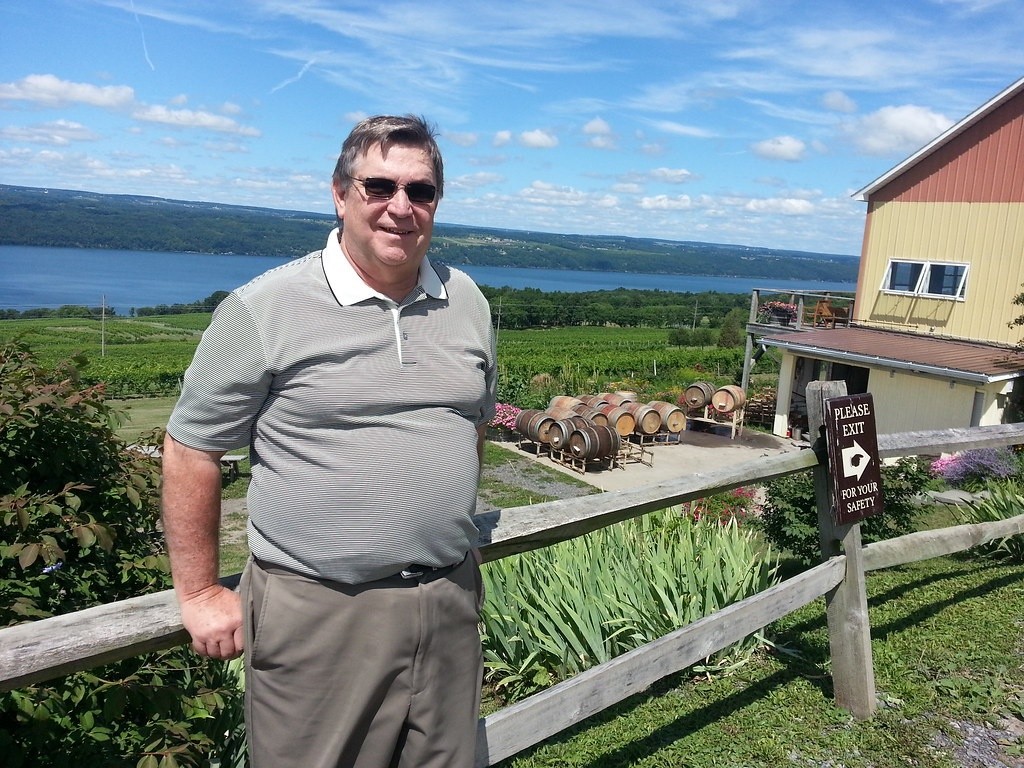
[768,309,790,326]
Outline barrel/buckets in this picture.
[647,401,685,432]
[548,417,597,450]
[576,395,609,408]
[550,396,588,411]
[597,404,635,436]
[684,381,717,408]
[515,410,555,444]
[712,385,746,412]
[545,407,579,421]
[570,426,621,459]
[571,405,609,426]
[621,403,661,435]
[597,393,632,407]
[615,391,637,403]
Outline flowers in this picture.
[762,302,797,319]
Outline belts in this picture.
[398,564,432,580]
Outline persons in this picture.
[160,114,496,767]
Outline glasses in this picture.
[344,173,438,204]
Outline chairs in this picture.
[817,300,848,329]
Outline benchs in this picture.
[220,454,248,481]
